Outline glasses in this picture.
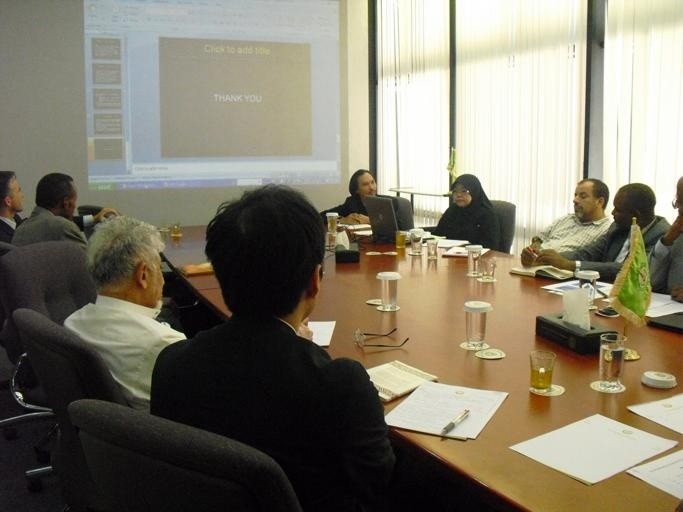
[354,327,408,349]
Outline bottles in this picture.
[171,225,183,246]
[158,229,169,246]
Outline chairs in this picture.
[489,200,516,255]
[394,197,414,231]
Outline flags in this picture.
[606,224,651,329]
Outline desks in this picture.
[160,226,683,512]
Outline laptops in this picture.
[362,193,434,245]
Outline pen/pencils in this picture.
[597,289,607,298]
[441,407,471,436]
[527,246,538,257]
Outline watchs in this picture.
[573,258,583,272]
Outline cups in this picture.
[378,271,398,310]
[328,212,338,232]
[411,231,423,253]
[528,350,555,394]
[483,257,496,280]
[396,230,409,249]
[578,270,596,306]
[464,300,486,347]
[599,334,627,393]
[467,244,482,274]
[426,239,438,260]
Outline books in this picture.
[364,360,438,402]
[509,263,573,281]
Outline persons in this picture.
[521,177,613,269]
[11,175,88,246]
[647,176,683,304]
[150,183,414,511]
[62,214,188,412]
[1,170,120,245]
[532,185,672,284]
[431,173,502,252]
[318,169,404,231]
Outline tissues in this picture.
[535,287,617,356]
[333,228,360,264]
